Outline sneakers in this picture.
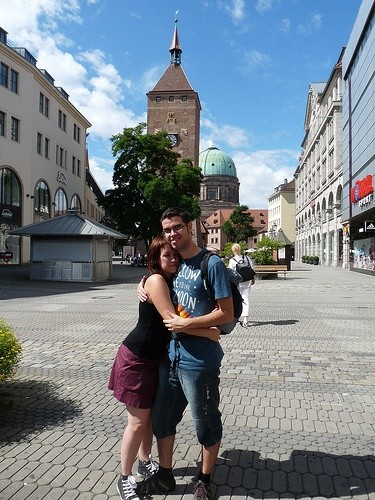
[192,480,211,500]
[137,472,177,495]
[116,473,140,500]
[138,454,159,478]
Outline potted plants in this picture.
[302,256,319,265]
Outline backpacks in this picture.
[231,255,256,282]
[201,251,244,335]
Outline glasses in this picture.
[163,222,188,235]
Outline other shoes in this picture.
[243,322,248,328]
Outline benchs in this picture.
[254,265,287,280]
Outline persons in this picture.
[359,245,365,260]
[136,207,234,500]
[229,243,255,327]
[126,251,147,267]
[368,245,373,261]
[108,236,221,500]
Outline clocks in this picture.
[165,134,177,147]
